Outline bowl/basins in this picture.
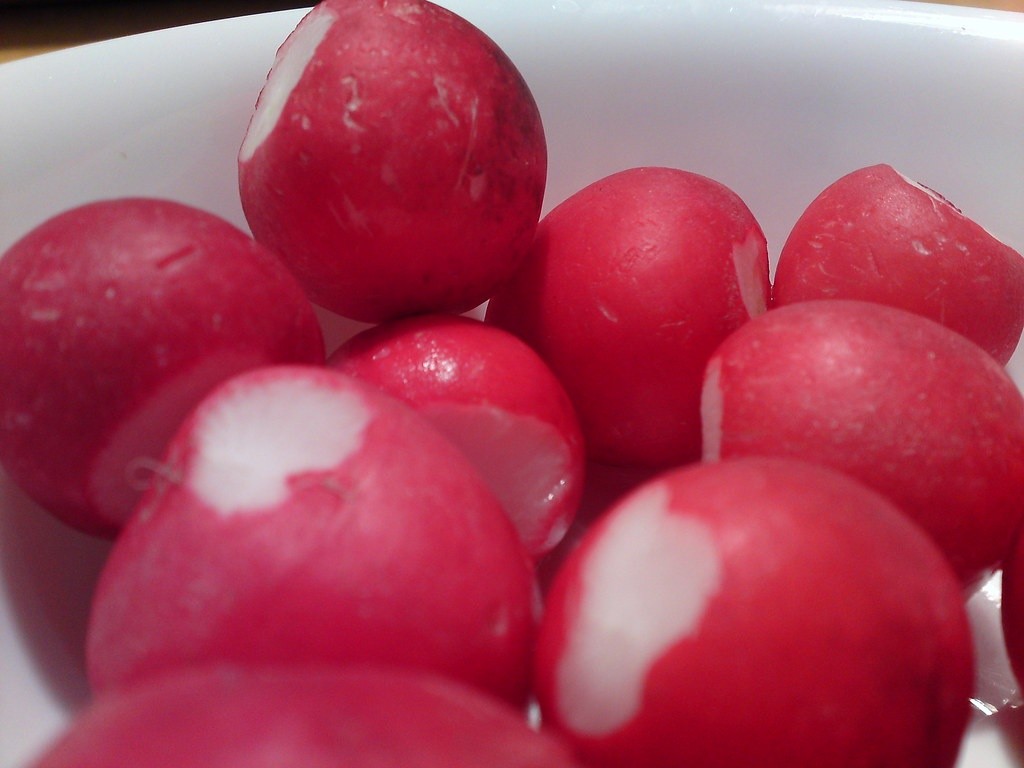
[0,0,1024,768]
